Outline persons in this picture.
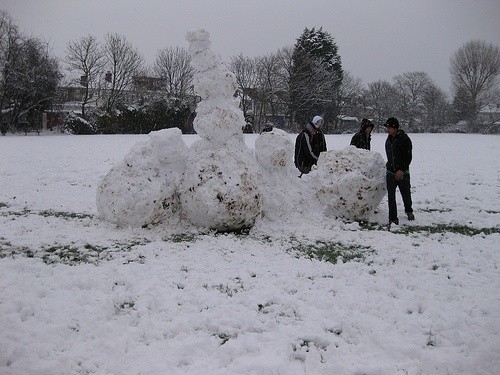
[351,118,375,151]
[383,117,414,225]
[294,122,320,178]
[311,116,327,157]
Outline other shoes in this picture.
[407,211,414,220]
[389,218,399,225]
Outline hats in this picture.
[313,116,324,123]
[383,117,400,129]
